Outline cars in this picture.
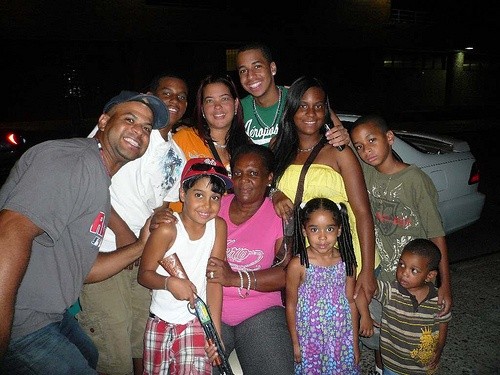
[334,113,487,236]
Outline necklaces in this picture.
[297,134,323,155]
[212,134,230,148]
[253,85,283,130]
[375,159,397,205]
[254,88,281,128]
[95,137,114,177]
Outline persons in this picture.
[78,44,450,375]
[0,90,170,375]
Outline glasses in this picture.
[199,71,231,84]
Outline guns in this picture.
[157,253,234,375]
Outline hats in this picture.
[104,90,170,130]
[181,157,234,190]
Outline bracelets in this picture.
[268,188,280,201]
[251,271,256,290]
[237,270,251,299]
[163,276,168,290]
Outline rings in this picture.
[210,271,214,279]
